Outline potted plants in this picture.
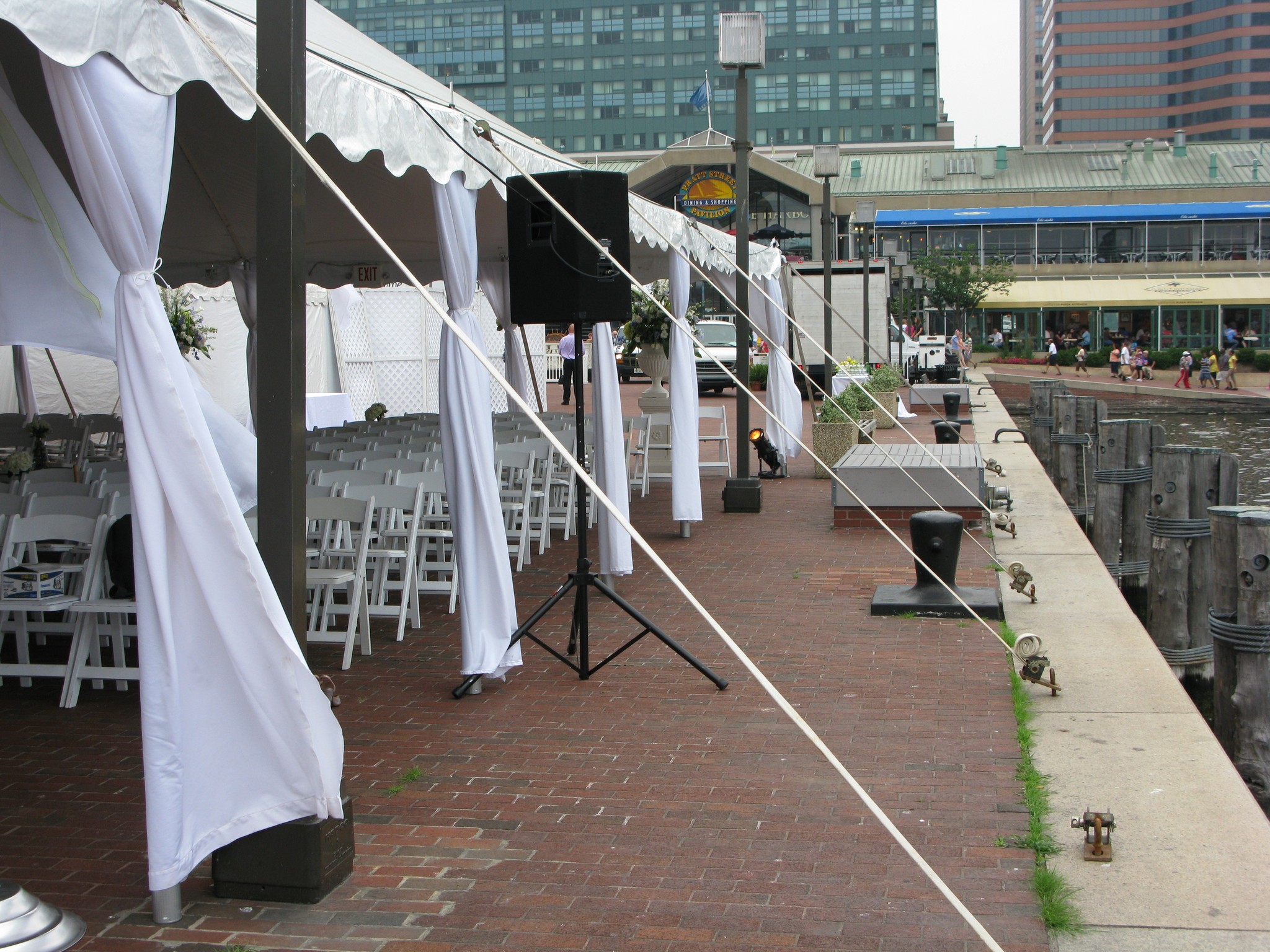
[812,362,904,479]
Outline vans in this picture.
[613,320,749,393]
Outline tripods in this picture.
[451,321,730,700]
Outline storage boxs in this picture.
[1,563,64,600]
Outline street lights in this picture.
[882,238,936,379]
[717,9,766,512]
[813,142,841,401]
[855,200,876,367]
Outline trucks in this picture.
[781,257,924,394]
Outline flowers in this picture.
[621,279,712,373]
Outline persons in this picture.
[749,330,769,356]
[1110,341,1156,382]
[612,330,617,344]
[586,332,593,342]
[1074,345,1091,377]
[558,324,585,406]
[1174,347,1239,391]
[945,329,977,370]
[970,317,1255,351]
[1040,339,1062,375]
[901,317,926,341]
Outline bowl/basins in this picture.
[0,879,88,952]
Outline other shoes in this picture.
[1198,385,1206,388]
[1209,385,1214,388]
[1213,385,1219,389]
[1042,371,1047,374]
[1136,378,1143,382]
[1149,376,1153,380]
[1225,387,1232,390]
[1174,385,1180,388]
[973,363,977,369]
[1056,373,1062,375]
[1232,388,1238,390]
[1185,387,1192,389]
[1087,375,1091,377]
[1111,375,1117,378]
[1118,375,1122,378]
[1122,378,1128,382]
[561,402,569,405]
[1074,375,1080,376]
[1143,377,1149,379]
[1127,377,1133,380]
[964,367,970,370]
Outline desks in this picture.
[1009,339,1025,352]
[1063,339,1077,348]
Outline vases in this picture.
[637,343,669,398]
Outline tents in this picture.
[0,0,802,924]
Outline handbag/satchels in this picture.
[1215,371,1222,382]
[1082,349,1088,359]
[1120,363,1132,376]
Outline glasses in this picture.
[613,334,617,336]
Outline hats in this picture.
[1182,351,1190,356]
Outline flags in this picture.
[689,79,712,112]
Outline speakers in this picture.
[506,168,634,324]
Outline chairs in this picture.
[0,406,740,711]
[950,250,1270,267]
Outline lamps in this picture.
[750,426,787,479]
